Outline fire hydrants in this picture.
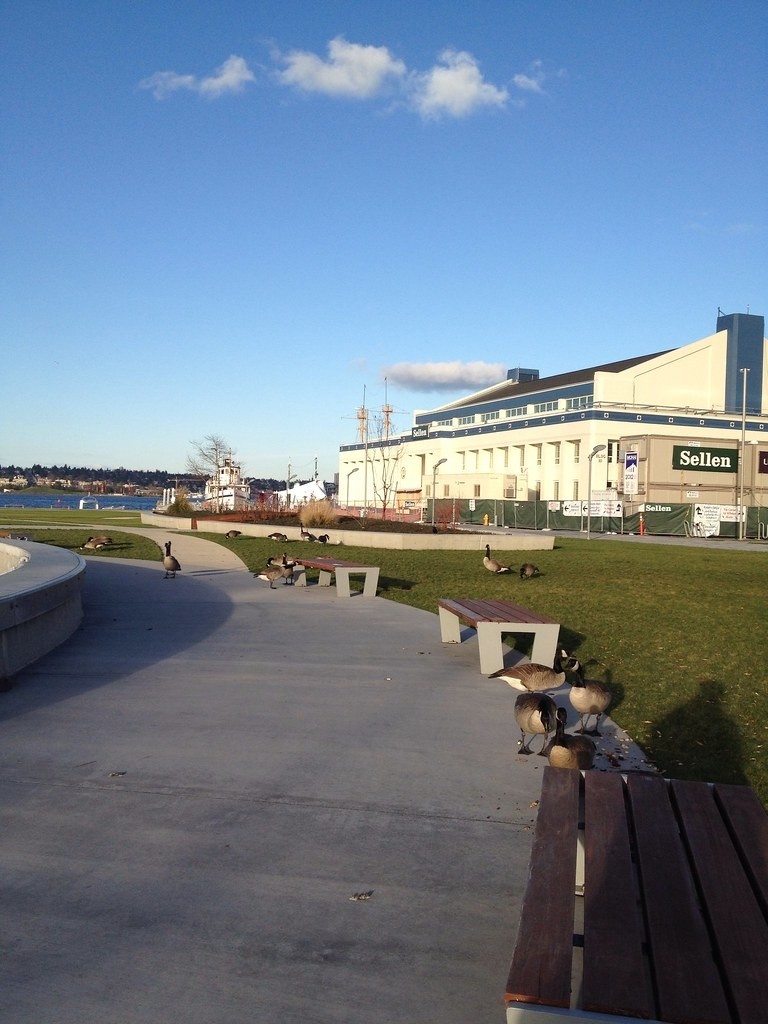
[482,513,489,526]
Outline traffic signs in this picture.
[582,500,623,517]
[694,503,747,523]
[562,500,581,517]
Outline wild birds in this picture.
[225,530,241,539]
[268,533,287,542]
[562,658,611,737]
[79,536,113,552]
[484,544,504,573]
[549,707,597,770]
[300,523,311,542]
[164,541,181,579]
[520,563,540,578]
[253,552,300,590]
[487,649,578,694]
[514,692,558,757]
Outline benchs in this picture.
[505,765,767,1024]
[434,596,560,675]
[289,559,379,600]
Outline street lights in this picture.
[245,478,255,511]
[346,466,360,514]
[738,368,751,542]
[431,458,448,526]
[286,474,298,511]
[586,443,606,540]
